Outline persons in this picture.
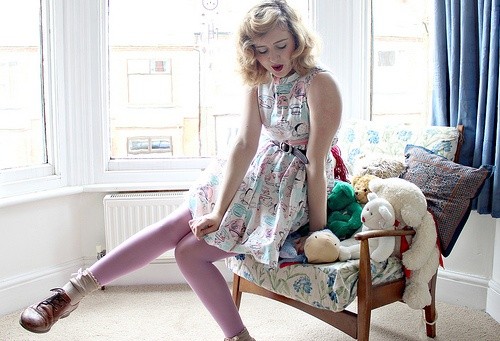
[18,0,345,341]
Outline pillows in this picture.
[399,144,496,257]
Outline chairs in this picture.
[233,120,465,341]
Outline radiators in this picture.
[103,190,192,264]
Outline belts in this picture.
[271,139,309,165]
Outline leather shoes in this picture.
[18,288,81,333]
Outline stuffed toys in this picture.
[303,159,440,309]
